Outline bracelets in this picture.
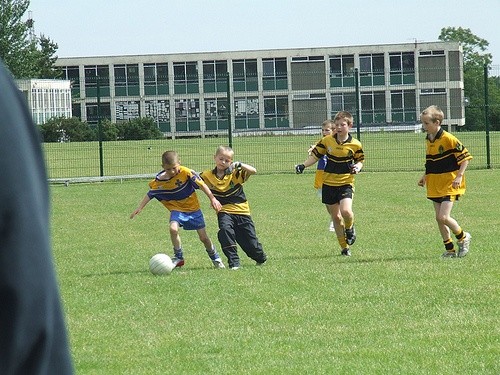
[208,193,214,200]
[456,174,464,178]
[235,162,242,171]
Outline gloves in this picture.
[226,161,241,174]
[296,164,305,174]
[350,166,360,174]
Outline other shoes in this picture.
[330,221,335,232]
[229,266,239,271]
[345,223,356,245]
[341,248,351,256]
[255,255,268,269]
[457,232,472,258]
[171,257,184,267]
[442,251,456,258]
[213,258,225,269]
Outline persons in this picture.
[308,119,336,231]
[0,60,74,375]
[417,104,472,259]
[295,111,365,257]
[196,146,268,270]
[129,150,227,270]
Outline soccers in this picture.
[148,253,172,276]
